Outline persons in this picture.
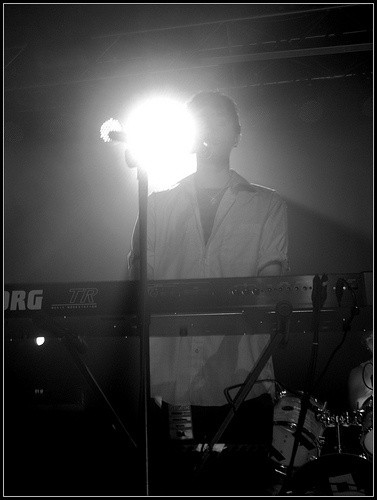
[348,330,374,417]
[118,89,296,453]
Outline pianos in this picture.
[0,272,377,337]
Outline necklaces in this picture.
[195,177,229,205]
